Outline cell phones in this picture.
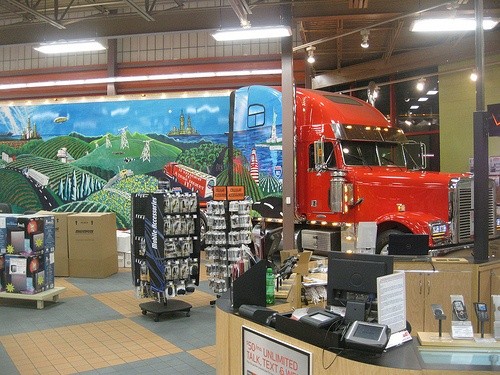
[475,302,489,323]
[453,301,468,322]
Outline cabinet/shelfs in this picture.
[394,262,500,334]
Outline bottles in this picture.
[266,268,275,304]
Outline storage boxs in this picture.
[35,210,70,276]
[0,214,55,295]
[68,213,118,279]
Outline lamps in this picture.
[360,29,370,48]
[306,47,316,63]
[209,0,292,42]
[33,0,109,55]
[409,0,500,30]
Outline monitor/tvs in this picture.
[388,234,429,256]
[344,320,388,348]
[327,251,394,322]
